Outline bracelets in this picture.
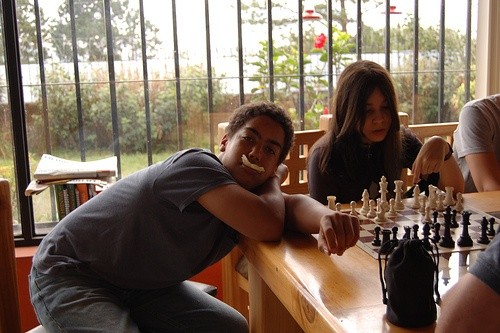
[444,142,453,161]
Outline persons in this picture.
[28,100,360,333]
[451,94,500,193]
[306,61,465,205]
[434,232,500,333]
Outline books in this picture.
[25,153,118,223]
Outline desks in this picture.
[238,190,500,333]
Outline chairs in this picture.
[0,179,21,333]
[397,112,460,199]
[218,114,340,323]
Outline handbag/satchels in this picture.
[376,236,442,329]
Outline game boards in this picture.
[324,175,500,261]
